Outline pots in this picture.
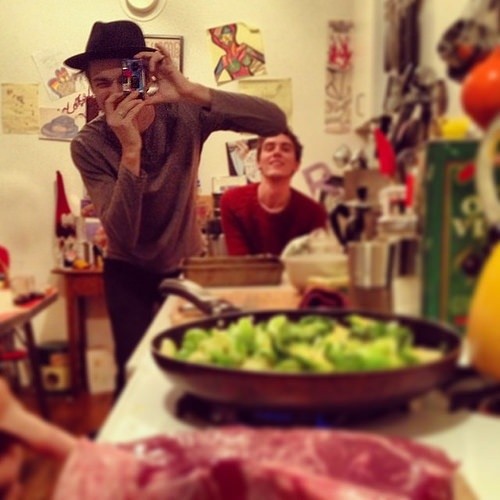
[151,278,463,412]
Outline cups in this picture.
[348,241,395,311]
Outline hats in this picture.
[63,20,157,69]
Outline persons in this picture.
[64,20,286,406]
[221,127,328,256]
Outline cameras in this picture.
[120,58,146,93]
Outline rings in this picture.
[116,109,125,117]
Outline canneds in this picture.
[419,140,500,330]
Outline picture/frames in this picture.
[143,34,185,73]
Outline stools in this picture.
[0,350,29,390]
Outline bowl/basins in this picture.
[280,260,348,292]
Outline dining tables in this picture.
[53,270,499,500]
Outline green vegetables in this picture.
[159,313,443,371]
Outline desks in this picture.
[0,289,59,420]
[50,261,112,395]
